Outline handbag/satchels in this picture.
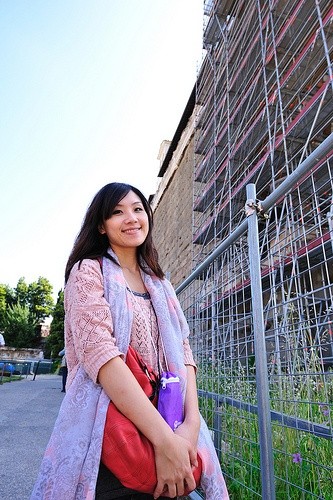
[101,345,203,496]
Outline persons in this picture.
[58,347,68,392]
[28,183,230,500]
